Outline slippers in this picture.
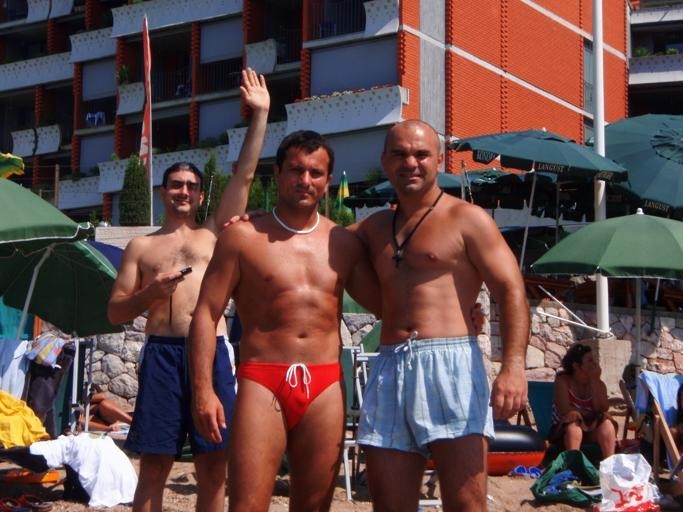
[527,467,542,478]
[0,498,34,512]
[17,494,52,511]
[508,465,529,477]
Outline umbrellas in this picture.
[326,169,559,226]
[446,126,629,274]
[526,205,683,383]
[0,175,135,340]
[601,112,683,208]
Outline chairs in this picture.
[340,343,369,501]
[512,368,683,502]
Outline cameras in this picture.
[169,266,193,282]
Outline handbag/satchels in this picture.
[531,449,601,506]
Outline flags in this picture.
[138,17,152,174]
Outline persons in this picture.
[544,342,619,460]
[105,67,271,511]
[187,129,486,511]
[223,118,531,512]
[622,363,683,503]
[76,382,132,432]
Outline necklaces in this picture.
[390,188,443,269]
[272,205,321,235]
